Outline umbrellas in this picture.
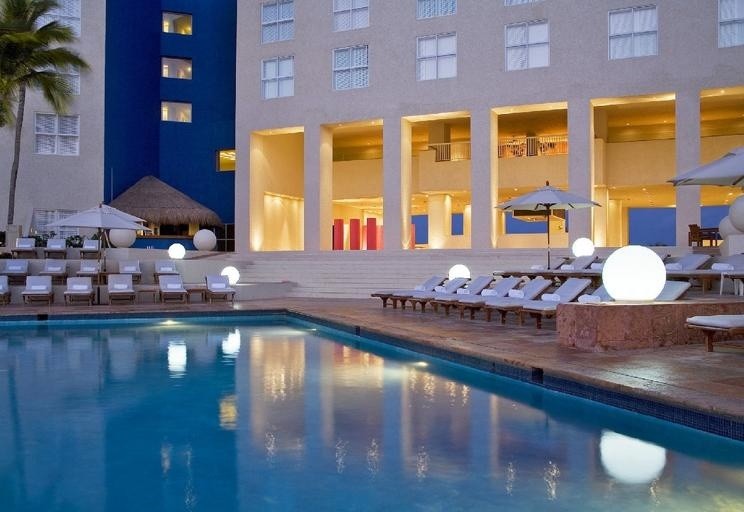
[666,147,743,186]
[494,181,602,269]
[45,202,151,285]
[59,202,147,271]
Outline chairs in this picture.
[370,246,743,353]
[688,224,703,247]
[0,236,237,307]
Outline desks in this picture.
[701,228,719,246]
